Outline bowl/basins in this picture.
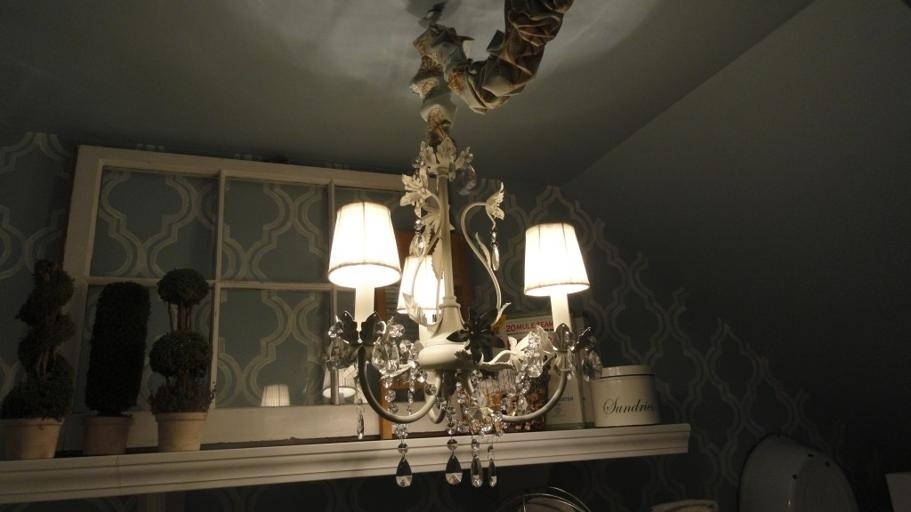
[735,429,861,512]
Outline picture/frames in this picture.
[489,308,599,433]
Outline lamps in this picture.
[321,2,604,488]
[323,364,356,406]
[259,383,290,409]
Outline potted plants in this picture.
[147,268,214,452]
[81,281,150,455]
[2,262,78,459]
